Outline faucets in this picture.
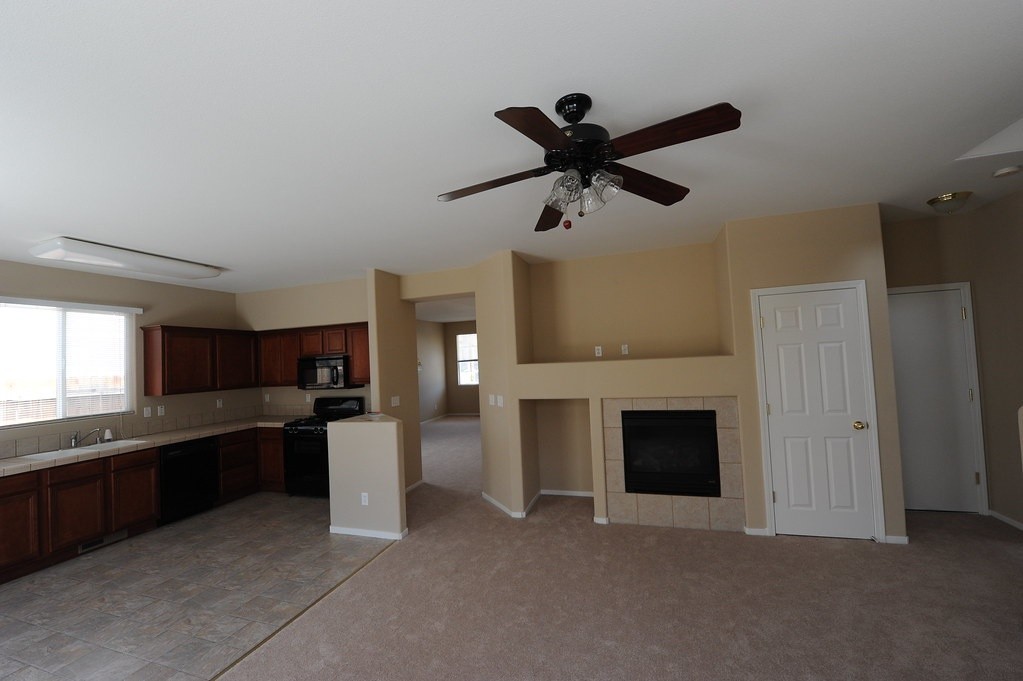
[70,428,100,448]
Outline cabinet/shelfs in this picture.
[140,318,368,399]
[0,427,283,587]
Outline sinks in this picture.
[79,441,144,451]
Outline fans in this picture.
[436,91,743,235]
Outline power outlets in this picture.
[143,406,152,418]
[305,393,311,402]
[594,345,603,357]
[157,406,165,417]
[216,399,223,408]
[264,394,269,402]
[621,344,629,354]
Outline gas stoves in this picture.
[283,397,366,428]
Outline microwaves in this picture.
[296,351,350,390]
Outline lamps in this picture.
[925,189,976,217]
[25,236,226,284]
[541,89,625,219]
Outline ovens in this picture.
[283,428,329,499]
